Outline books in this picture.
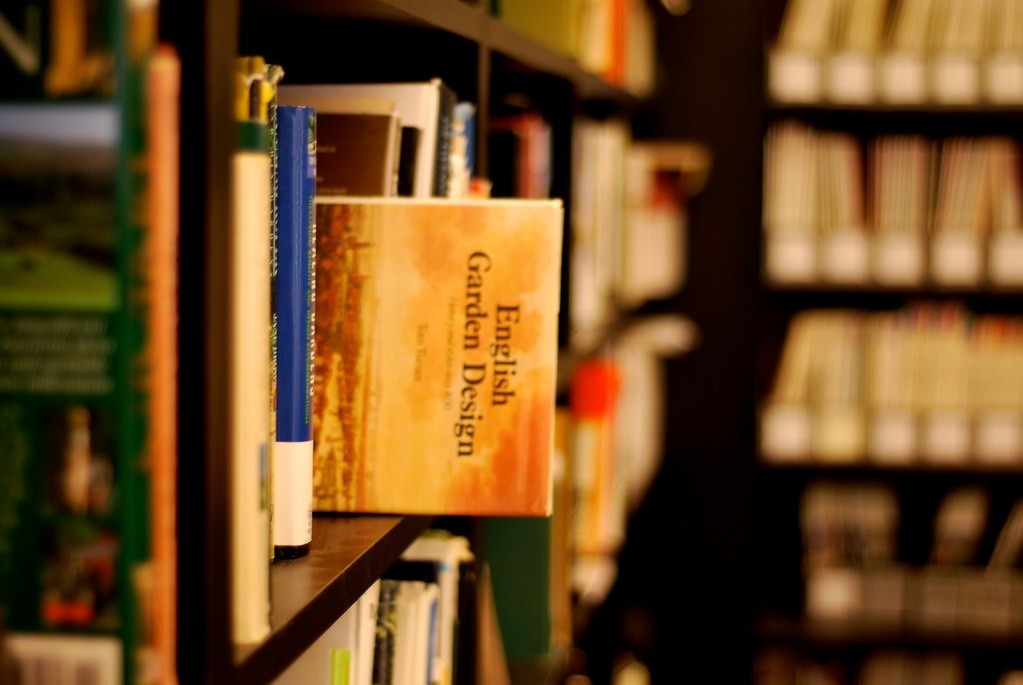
[762,0,1023,631]
[1,2,691,684]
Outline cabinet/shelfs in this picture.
[0,0,1023,685]
